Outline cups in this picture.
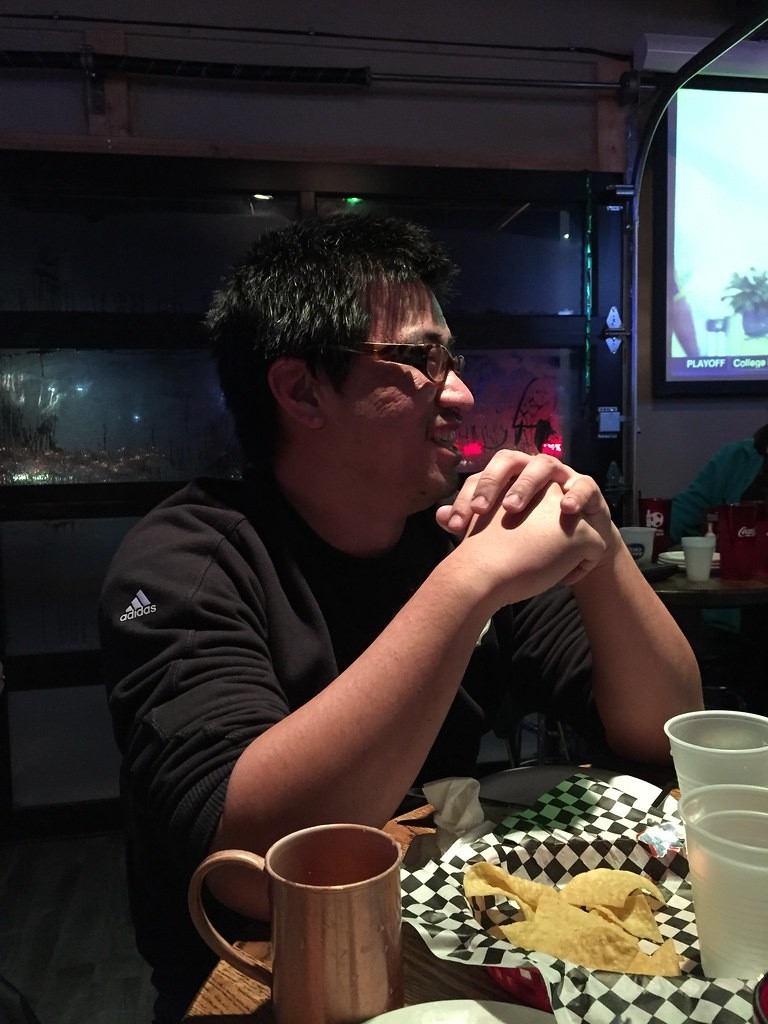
[189,823,404,1024]
[743,501,768,578]
[705,507,718,531]
[681,537,716,582]
[664,710,768,796]
[620,527,658,563]
[638,498,673,551]
[677,784,768,979]
[718,504,755,579]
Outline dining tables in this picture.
[179,789,683,1024]
[649,572,768,608]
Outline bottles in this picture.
[706,523,716,537]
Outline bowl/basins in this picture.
[499,314,587,347]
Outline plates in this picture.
[657,551,721,571]
[364,999,557,1024]
[436,767,678,854]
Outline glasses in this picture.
[320,340,469,387]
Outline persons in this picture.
[662,423,768,552]
[97,203,705,1024]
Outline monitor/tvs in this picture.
[649,74,768,401]
